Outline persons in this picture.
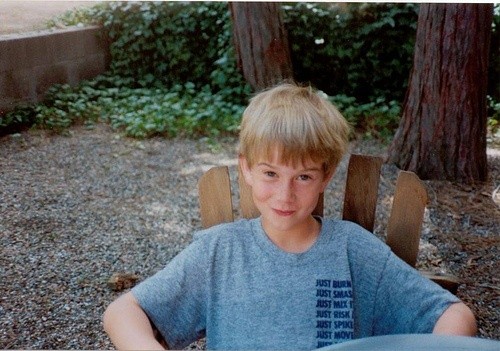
[102,80,476,350]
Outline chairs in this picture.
[200,152,459,297]
